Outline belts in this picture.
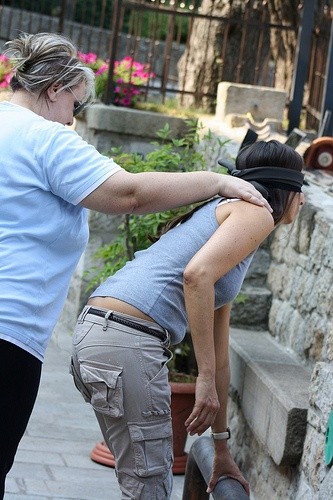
[84,308,166,343]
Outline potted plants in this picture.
[81,119,239,478]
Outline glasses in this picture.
[68,87,90,117]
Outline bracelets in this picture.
[210,426,232,439]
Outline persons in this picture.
[0,32,274,500]
[67,139,305,500]
